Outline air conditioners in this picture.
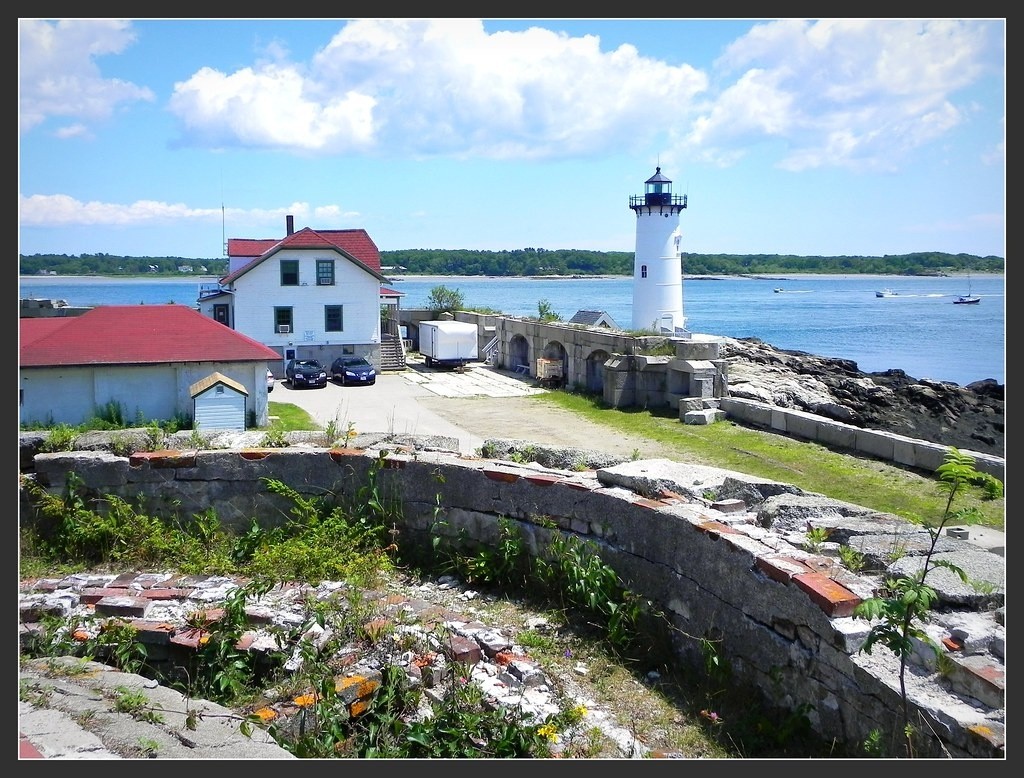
[321,278,330,284]
[279,325,289,332]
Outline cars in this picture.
[331,355,376,386]
[286,359,327,389]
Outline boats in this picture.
[774,286,784,293]
[875,288,899,298]
[953,295,981,304]
